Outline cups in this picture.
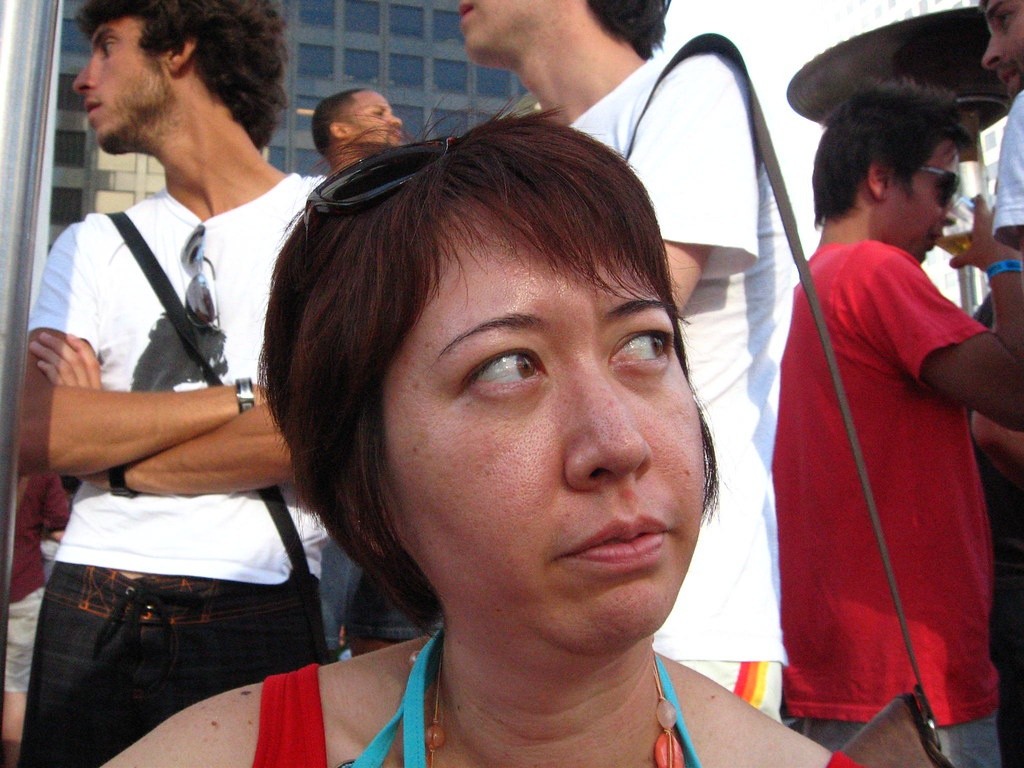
[932,197,976,256]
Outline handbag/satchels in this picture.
[839,693,953,768]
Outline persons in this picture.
[771,1,1024,768]
[310,89,402,179]
[460,0,792,725]
[98,115,834,768]
[0,0,336,768]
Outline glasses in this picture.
[918,165,960,207]
[180,225,220,330]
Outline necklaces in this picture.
[425,648,685,767]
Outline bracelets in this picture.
[235,377,254,414]
[109,465,142,499]
[986,258,1023,282]
